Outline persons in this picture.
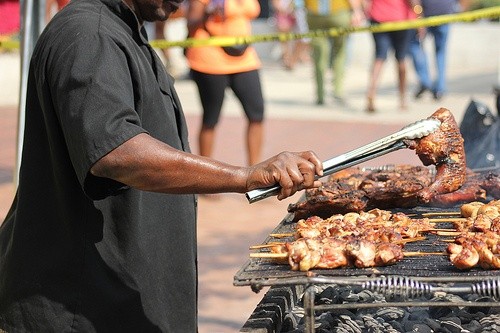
[268,0,306,68]
[184,0,266,167]
[1,0,323,333]
[304,0,360,105]
[361,0,426,112]
[154,20,175,68]
[407,0,453,100]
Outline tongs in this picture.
[245,117,441,204]
